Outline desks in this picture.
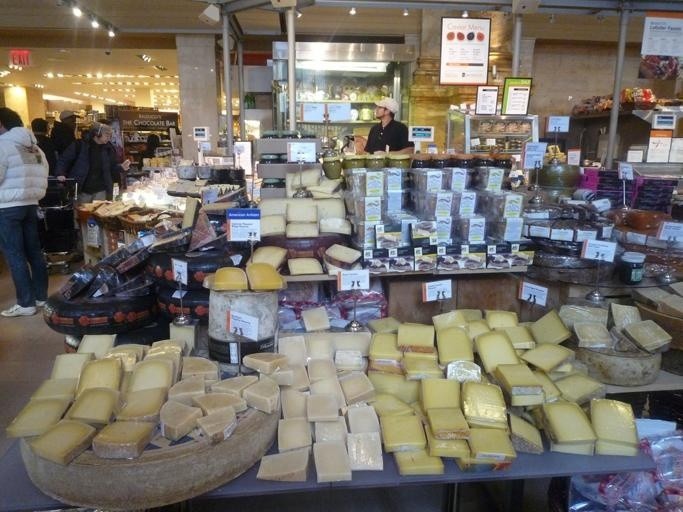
[0,369,644,512]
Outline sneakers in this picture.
[36,300,46,307]
[1,304,37,317]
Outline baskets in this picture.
[92,206,141,231]
[74,203,110,222]
[116,209,161,234]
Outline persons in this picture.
[363,97,415,159]
[0,105,49,319]
[31,109,163,275]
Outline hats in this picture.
[59,110,80,120]
[374,97,399,114]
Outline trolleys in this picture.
[33,177,82,275]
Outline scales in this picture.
[193,126,234,166]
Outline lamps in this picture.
[63,1,121,40]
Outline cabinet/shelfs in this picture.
[120,127,178,179]
[284,97,382,126]
[203,267,525,368]
[446,109,540,154]
